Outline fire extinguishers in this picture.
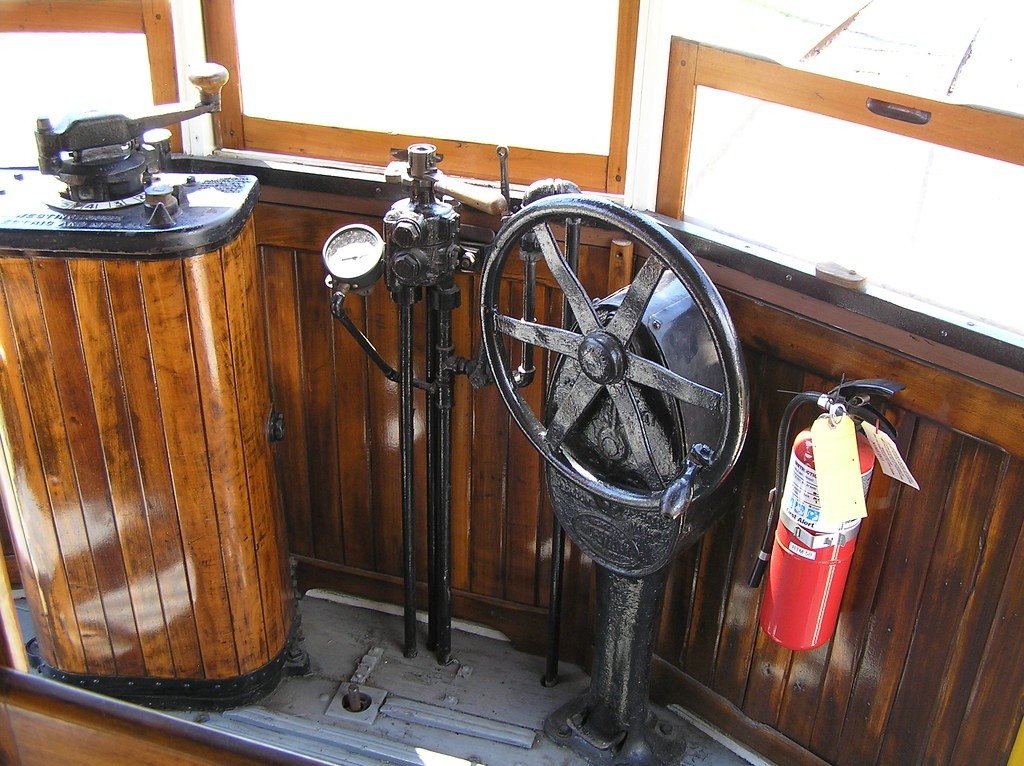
[746,375,910,652]
